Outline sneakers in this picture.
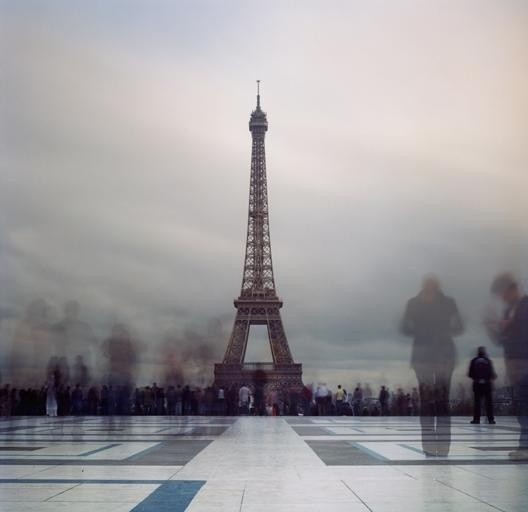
[470,420,480,424]
[489,420,496,425]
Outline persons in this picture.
[483,273,528,462]
[2,382,422,419]
[399,272,464,462]
[467,345,500,426]
[251,361,266,417]
